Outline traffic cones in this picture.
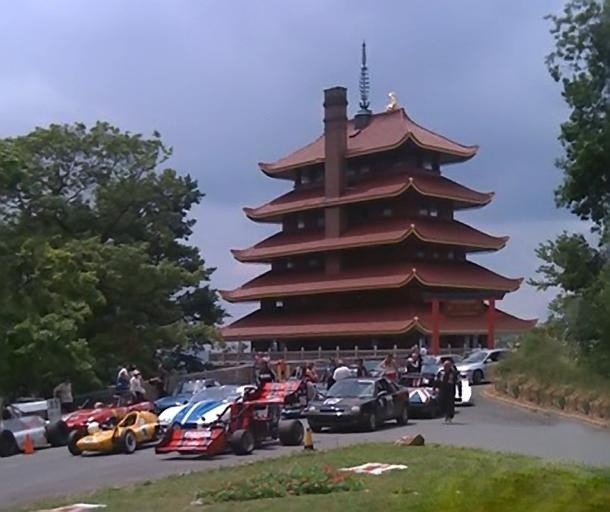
[303,428,314,450]
[23,433,33,453]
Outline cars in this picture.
[306,377,411,431]
[309,346,518,411]
[151,377,222,414]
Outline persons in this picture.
[53,375,74,413]
[114,361,187,404]
[432,361,462,420]
[252,347,423,383]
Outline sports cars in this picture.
[156,383,259,438]
